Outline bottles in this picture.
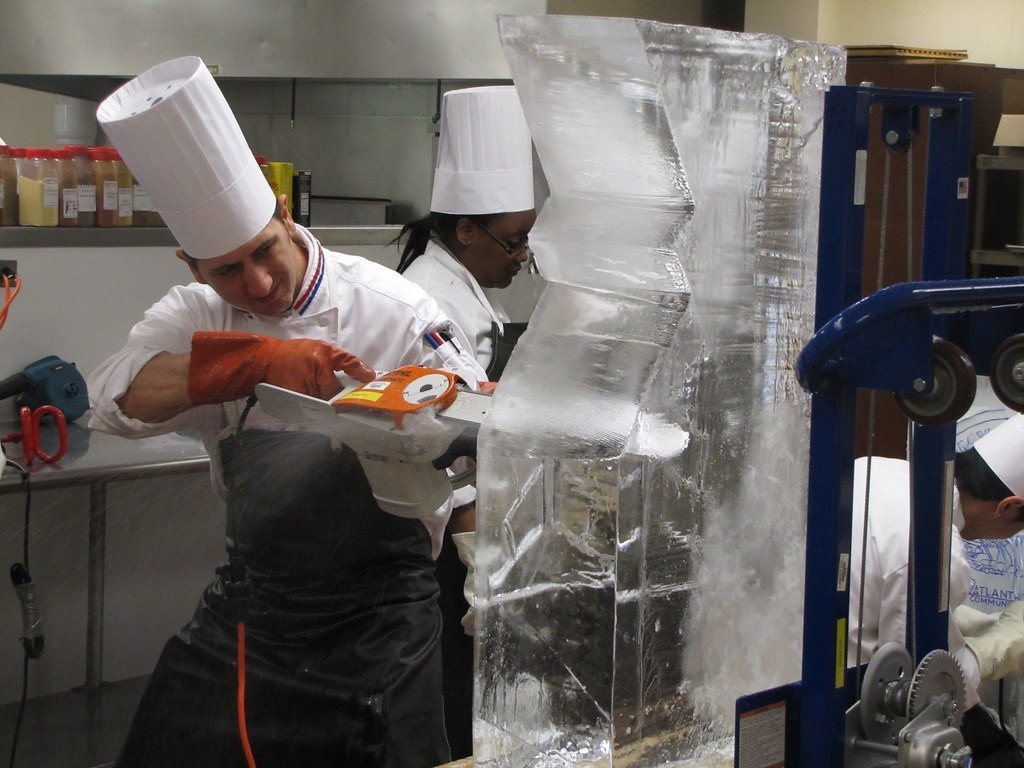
[255,155,313,228]
[0,144,137,228]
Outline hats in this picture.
[973,411,1024,498]
[431,86,536,215]
[96,56,276,259]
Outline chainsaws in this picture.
[255,364,691,521]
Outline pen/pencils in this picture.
[424,321,461,355]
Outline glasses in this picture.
[470,217,529,259]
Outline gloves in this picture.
[954,605,1003,636]
[965,598,1024,681]
[187,333,376,404]
[452,532,482,636]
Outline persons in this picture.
[846,412,1024,692]
[85,57,498,767]
[397,85,535,761]
